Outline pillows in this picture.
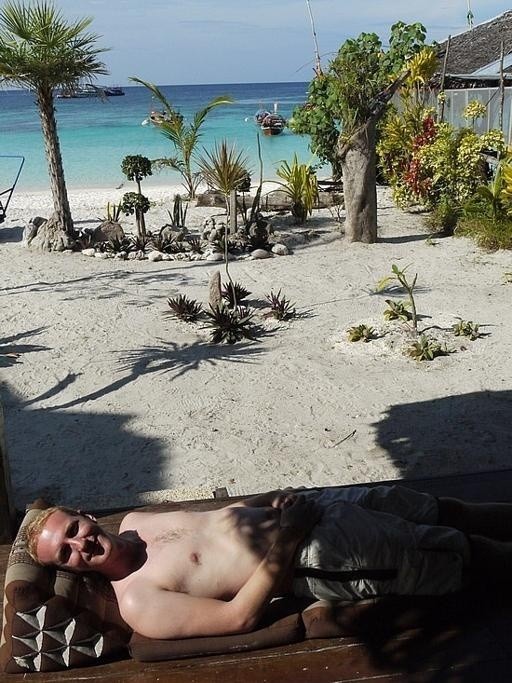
[1,498,132,674]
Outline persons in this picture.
[26,484,511,641]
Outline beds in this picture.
[0,470,512,682]
